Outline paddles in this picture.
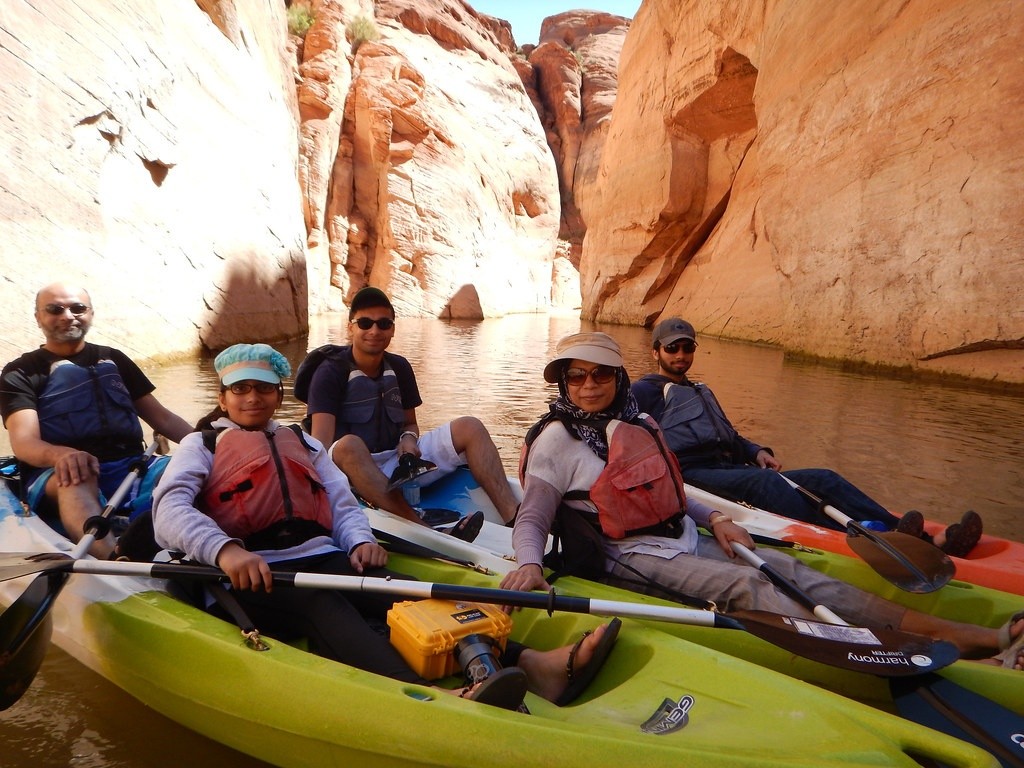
[0,442,160,712]
[732,539,1024,768]
[0,550,962,678]
[764,464,957,594]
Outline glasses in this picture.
[225,384,278,394]
[559,366,617,386]
[38,303,91,315]
[660,344,697,353]
[350,317,396,330]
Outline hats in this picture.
[214,344,291,386]
[652,318,699,346]
[351,287,392,311]
[544,332,624,383]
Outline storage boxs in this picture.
[386,597,513,679]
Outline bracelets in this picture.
[400,431,418,441]
[710,516,732,527]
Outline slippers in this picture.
[443,511,484,543]
[992,637,1024,670]
[556,617,622,706]
[998,611,1024,649]
[459,668,526,711]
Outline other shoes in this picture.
[947,510,983,558]
[899,510,924,539]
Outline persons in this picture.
[151,344,622,706]
[633,325,982,557]
[294,287,521,543]
[0,282,194,561]
[499,331,1024,669]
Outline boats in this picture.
[0,453,1002,767]
[347,470,1024,767]
[682,466,1024,599]
